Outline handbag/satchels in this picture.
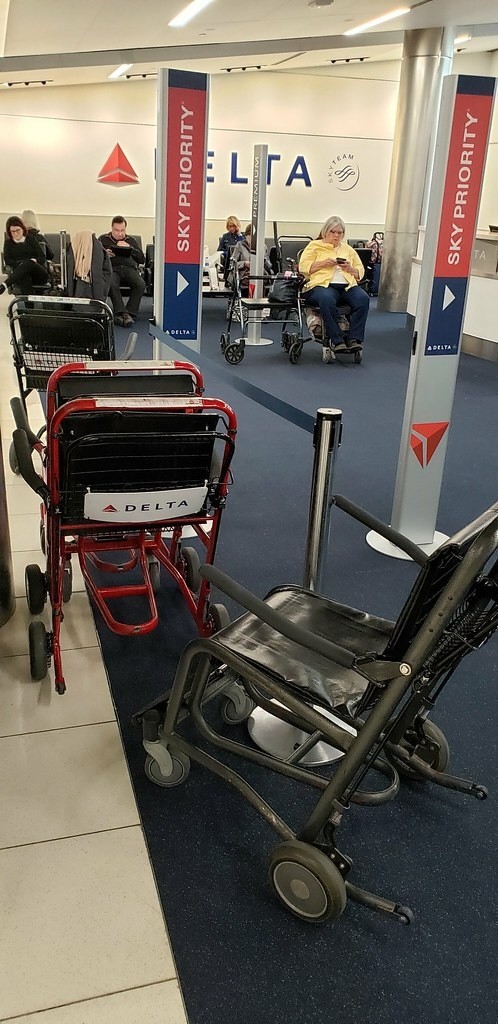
[267,271,298,303]
[365,236,384,264]
[226,297,248,322]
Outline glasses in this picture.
[10,229,21,233]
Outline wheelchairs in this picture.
[218,236,375,366]
[131,487,498,928]
[3,293,140,421]
[8,357,232,696]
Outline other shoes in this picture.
[0,284,5,295]
[114,315,123,326]
[349,339,363,353]
[335,343,347,352]
[123,314,135,328]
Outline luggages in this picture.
[368,232,384,296]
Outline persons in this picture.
[216,214,254,294]
[230,223,275,327]
[297,214,371,353]
[0,216,50,313]
[97,215,146,326]
[20,210,55,296]
